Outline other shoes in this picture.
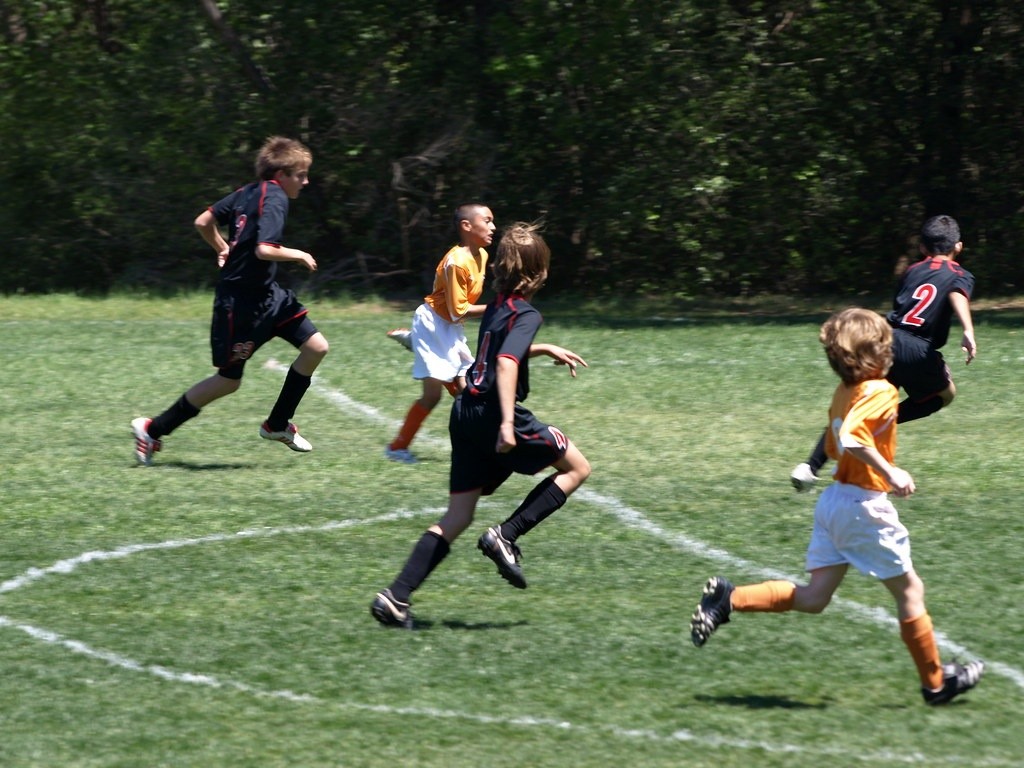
[791,462,818,494]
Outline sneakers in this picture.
[387,328,412,351]
[373,589,414,628]
[922,660,985,707]
[260,419,313,452]
[130,417,162,467]
[692,576,736,647]
[477,525,527,589]
[385,445,418,464]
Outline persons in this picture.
[130,137,328,466]
[789,214,976,488]
[386,203,496,465]
[369,225,592,628]
[691,308,985,702]
